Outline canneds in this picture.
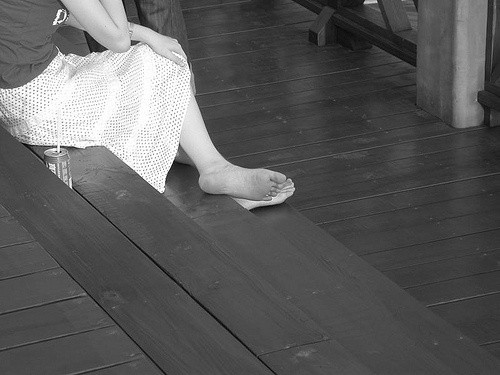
[43,148,73,190]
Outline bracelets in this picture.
[128,22,134,39]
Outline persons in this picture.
[1,1,295,211]
